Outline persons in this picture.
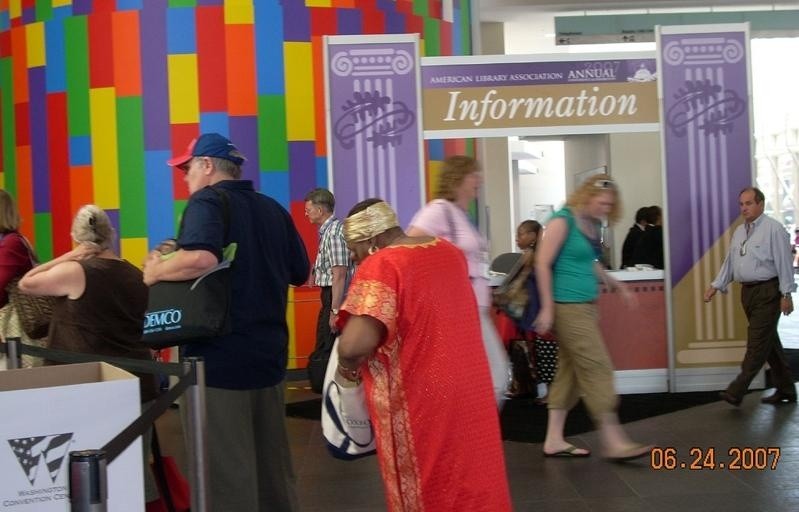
[699,187,798,409]
[535,173,660,464]
[143,133,312,512]
[18,204,162,510]
[0,189,39,311]
[325,196,514,511]
[301,155,666,412]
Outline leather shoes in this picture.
[762,394,796,403]
[720,393,740,407]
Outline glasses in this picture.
[593,181,615,189]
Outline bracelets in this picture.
[782,294,792,299]
[338,360,364,372]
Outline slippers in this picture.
[544,446,591,457]
[599,442,661,462]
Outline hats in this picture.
[167,133,243,166]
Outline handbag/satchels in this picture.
[1,275,66,370]
[140,269,233,351]
[308,334,335,394]
[493,241,540,331]
[321,335,378,460]
